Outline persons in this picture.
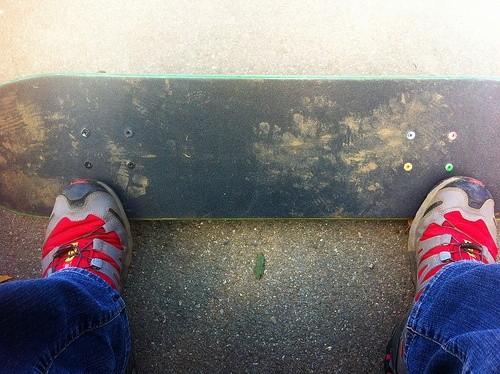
[0,176,500,374]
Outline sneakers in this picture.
[40,178,133,295]
[384,176,500,374]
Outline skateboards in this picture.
[0,74,500,221]
